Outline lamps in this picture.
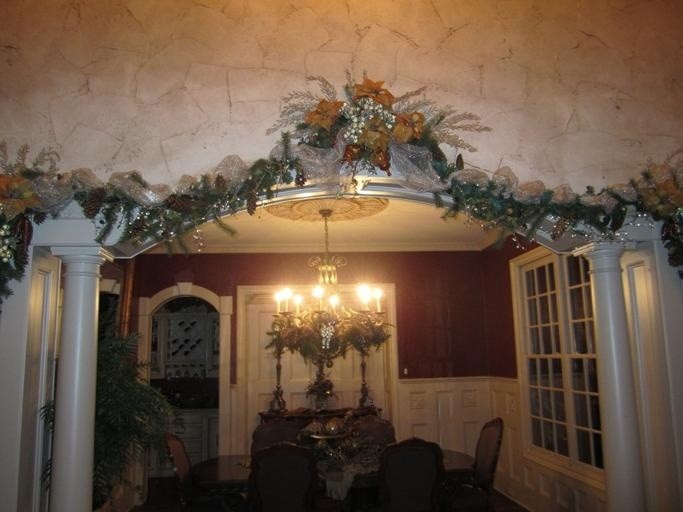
[264,208,395,367]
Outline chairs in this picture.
[249,439,316,511]
[454,417,502,511]
[164,430,193,509]
[377,437,443,510]
